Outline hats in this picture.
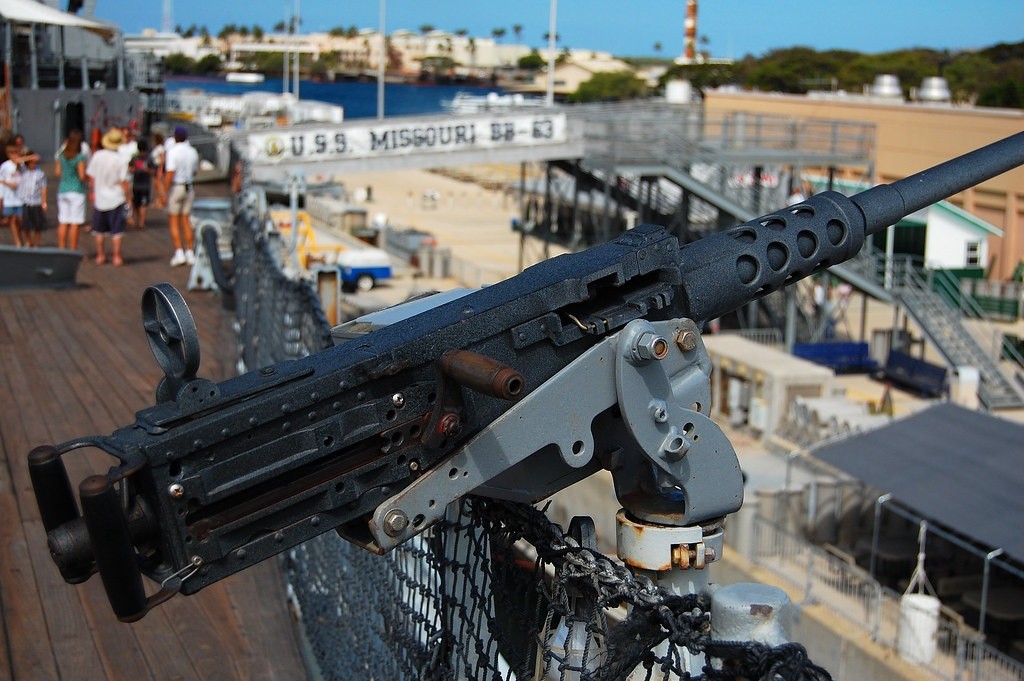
[175,126,188,139]
[102,129,127,150]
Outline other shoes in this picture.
[170,255,186,266]
[185,253,196,265]
[113,258,123,266]
[96,256,105,265]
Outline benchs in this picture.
[940,575,1001,604]
[837,524,872,553]
[820,544,874,595]
[939,602,987,660]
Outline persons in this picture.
[86,129,134,265]
[0,125,165,250]
[161,127,196,267]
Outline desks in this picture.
[963,585,1024,655]
[859,537,932,586]
